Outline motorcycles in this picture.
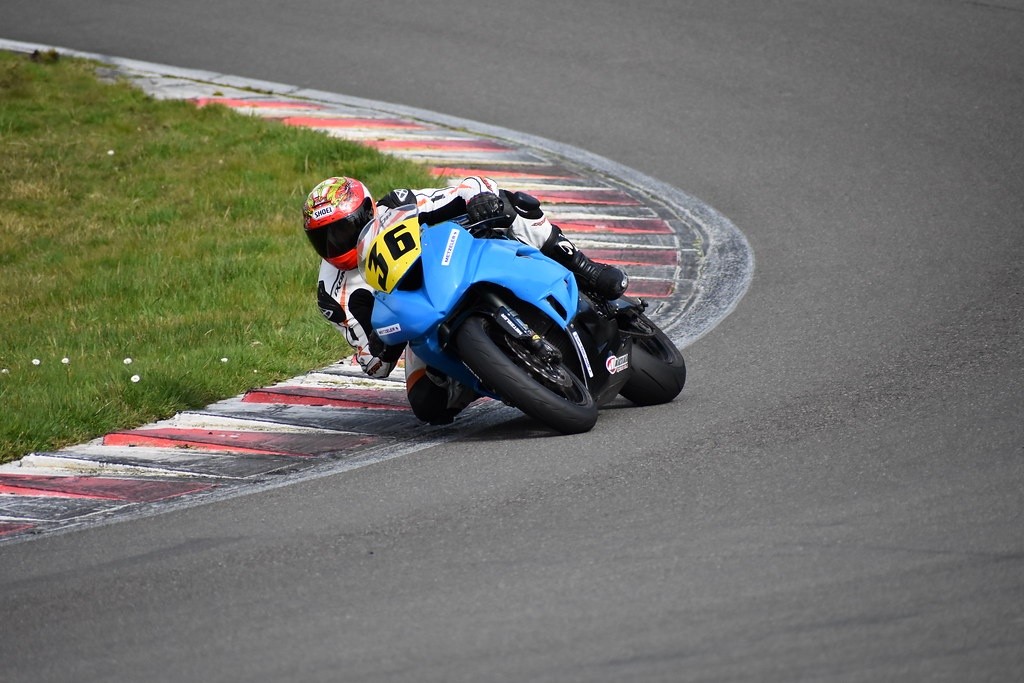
[347,202,688,435]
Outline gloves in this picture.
[369,330,408,362]
[466,192,504,224]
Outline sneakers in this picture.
[572,250,629,300]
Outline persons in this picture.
[301,175,628,425]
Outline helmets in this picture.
[303,176,378,271]
